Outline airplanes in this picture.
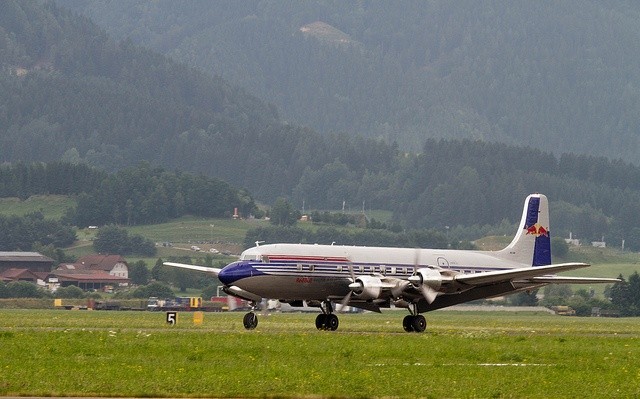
[163,192,624,331]
